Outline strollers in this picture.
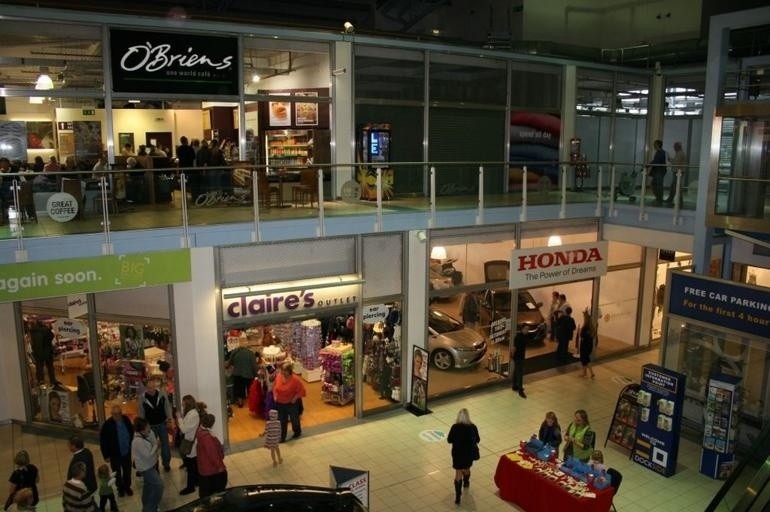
[612,166,648,202]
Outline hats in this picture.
[269,409,279,417]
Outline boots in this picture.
[453,477,462,505]
[463,472,471,488]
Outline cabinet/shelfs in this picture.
[265,130,315,177]
[57,335,92,374]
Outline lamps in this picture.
[29,67,53,104]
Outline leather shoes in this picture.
[513,385,528,398]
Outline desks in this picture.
[494,448,616,512]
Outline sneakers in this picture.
[161,460,171,472]
[118,486,134,497]
[179,486,196,495]
[280,431,301,444]
[581,373,597,378]
[272,458,284,465]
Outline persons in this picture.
[62,377,228,512]
[539,411,562,459]
[563,409,594,465]
[47,391,64,424]
[5,449,40,511]
[224,347,259,408]
[0,136,233,225]
[259,410,283,468]
[663,141,686,204]
[590,449,605,475]
[614,403,638,449]
[5,487,37,512]
[412,350,427,409]
[458,291,480,330]
[29,316,62,386]
[645,139,666,204]
[447,408,480,506]
[125,326,140,359]
[511,322,529,398]
[272,363,306,444]
[549,291,595,379]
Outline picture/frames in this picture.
[411,375,428,412]
[412,345,430,382]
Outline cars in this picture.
[429,254,462,302]
[384,304,489,372]
[470,259,549,350]
[165,481,369,512]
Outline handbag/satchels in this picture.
[471,441,480,460]
[178,436,195,456]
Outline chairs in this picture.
[607,468,623,512]
[63,180,82,220]
[94,181,120,217]
[249,166,319,209]
[17,181,38,223]
